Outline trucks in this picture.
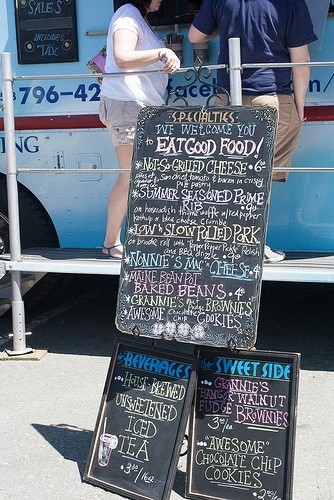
[0,0,334,302]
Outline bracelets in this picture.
[157,48,167,59]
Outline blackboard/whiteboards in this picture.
[115,106,277,350]
[83,337,200,500]
[190,344,301,500]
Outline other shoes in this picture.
[261,244,286,264]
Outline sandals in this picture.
[101,244,124,260]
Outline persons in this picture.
[99,0,181,262]
[188,0,319,265]
